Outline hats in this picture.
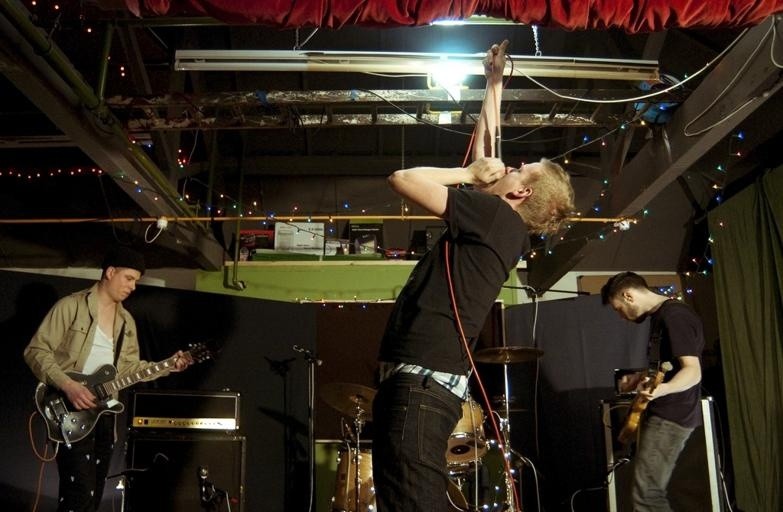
[101,247,145,275]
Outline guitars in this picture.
[34,343,211,443]
[618,370,664,443]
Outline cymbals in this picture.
[317,380,380,420]
[474,346,540,365]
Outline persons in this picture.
[602,268,708,511]
[21,246,196,511]
[367,36,573,511]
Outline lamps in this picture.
[174,24,664,84]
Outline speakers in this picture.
[127,387,241,432]
[124,428,247,512]
[602,397,721,512]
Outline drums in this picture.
[444,460,480,480]
[446,399,490,462]
[331,448,377,512]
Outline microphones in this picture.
[292,344,323,367]
